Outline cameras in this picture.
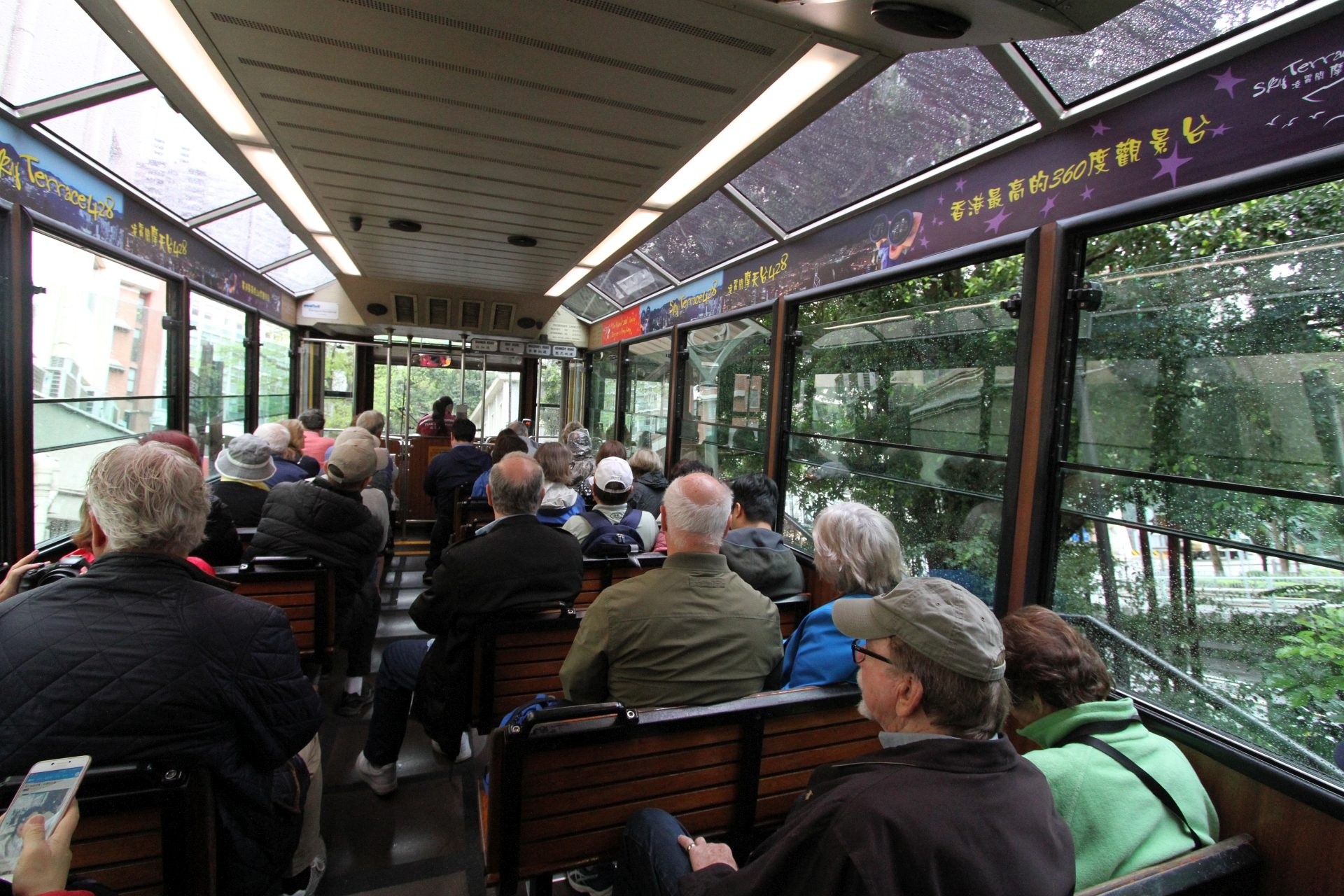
[16,555,90,594]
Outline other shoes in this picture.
[384,543,395,554]
[370,581,383,607]
[566,866,614,896]
[432,731,472,762]
[355,751,399,794]
[338,682,375,716]
[282,836,328,896]
[422,571,433,582]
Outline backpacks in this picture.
[480,694,580,797]
[572,508,645,559]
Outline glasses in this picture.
[568,462,576,469]
[851,639,896,664]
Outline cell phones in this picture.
[0,754,91,875]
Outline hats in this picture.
[815,461,850,480]
[334,426,381,448]
[327,438,389,484]
[594,456,634,493]
[214,432,277,481]
[592,437,602,449]
[831,576,1007,681]
[567,431,591,455]
[936,456,966,484]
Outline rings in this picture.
[687,843,696,851]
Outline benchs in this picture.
[455,515,494,537]
[489,592,816,705]
[454,496,494,519]
[557,544,670,604]
[207,554,339,669]
[464,681,891,896]
[0,755,219,896]
[454,481,476,499]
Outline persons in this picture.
[870,206,923,271]
[0,441,328,896]
[0,797,95,896]
[137,407,913,796]
[641,306,659,334]
[601,413,1003,602]
[419,353,446,367]
[567,578,1075,896]
[0,549,51,603]
[1000,603,1219,892]
[223,269,247,299]
[416,396,456,436]
